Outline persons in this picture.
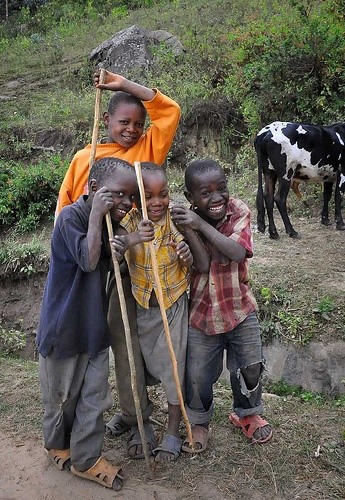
[54,67,181,458]
[169,158,272,452]
[106,161,194,463]
[35,157,137,490]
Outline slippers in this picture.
[105,413,130,436]
[229,412,273,444]
[152,434,182,460]
[70,455,125,489]
[128,423,156,458]
[44,447,71,472]
[181,425,213,453]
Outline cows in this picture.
[251,120,345,241]
